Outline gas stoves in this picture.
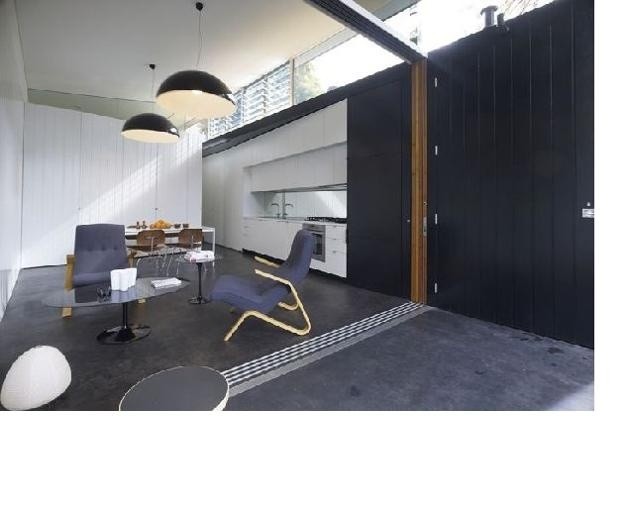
[304,216,347,224]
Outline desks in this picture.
[125,225,215,269]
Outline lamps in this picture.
[119,2,238,144]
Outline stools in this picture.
[174,253,223,304]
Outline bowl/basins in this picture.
[183,224,188,228]
[175,224,180,229]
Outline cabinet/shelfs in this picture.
[241,99,348,168]
[325,225,346,280]
[249,143,347,193]
[243,219,302,261]
[22,102,202,270]
[201,143,242,252]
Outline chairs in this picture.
[62,224,145,319]
[210,230,318,342]
[137,228,207,275]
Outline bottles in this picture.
[142,221,146,229]
[136,222,140,229]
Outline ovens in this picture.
[302,224,326,262]
